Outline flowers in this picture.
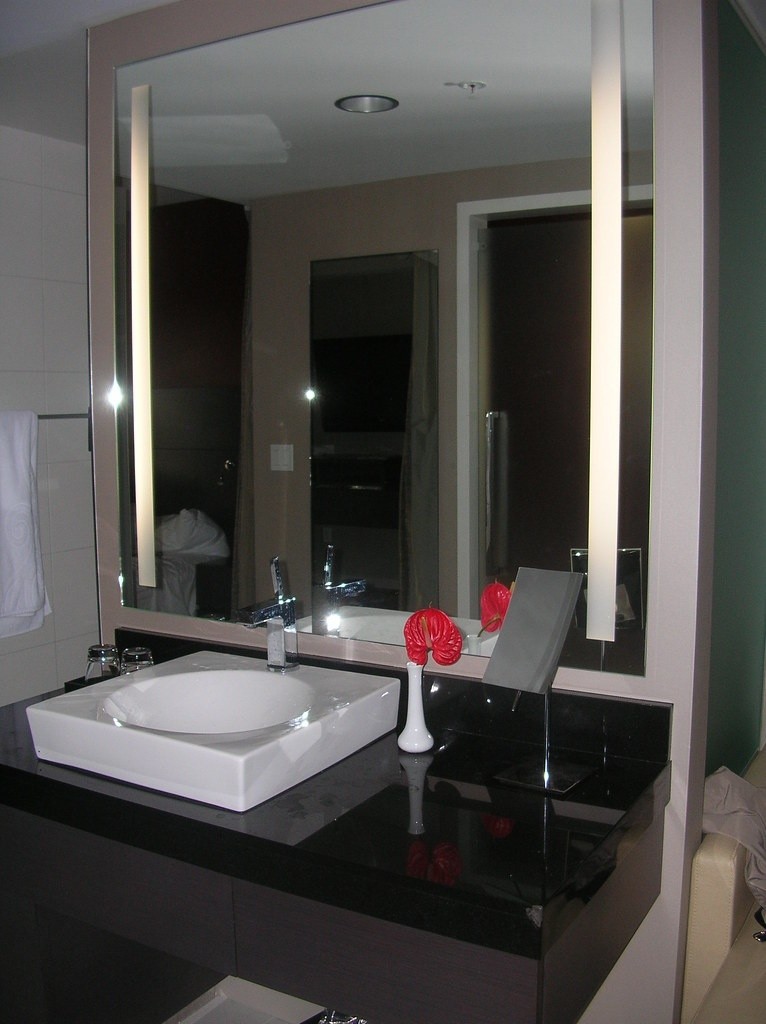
[403,607,462,666]
[478,581,511,638]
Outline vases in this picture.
[467,634,483,655]
[398,660,435,752]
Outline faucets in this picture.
[236,553,301,673]
[308,542,366,638]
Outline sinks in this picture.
[25,650,402,816]
[286,603,508,654]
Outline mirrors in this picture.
[109,0,653,679]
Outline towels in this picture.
[0,410,52,641]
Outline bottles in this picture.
[398,752,434,835]
[398,662,434,754]
[84,644,121,685]
[120,647,154,676]
[466,634,482,656]
